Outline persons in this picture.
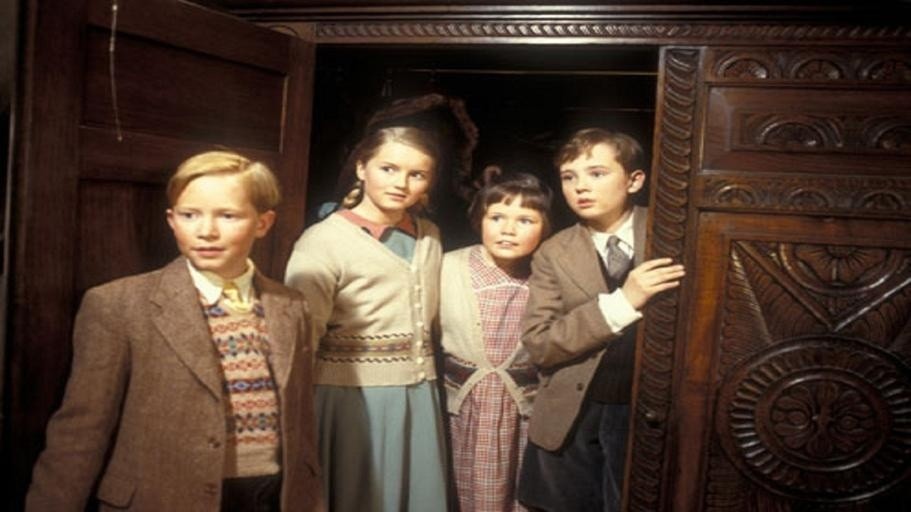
[282,118,451,511]
[24,150,325,511]
[437,167,556,511]
[518,125,686,511]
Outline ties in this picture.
[607,234,631,279]
